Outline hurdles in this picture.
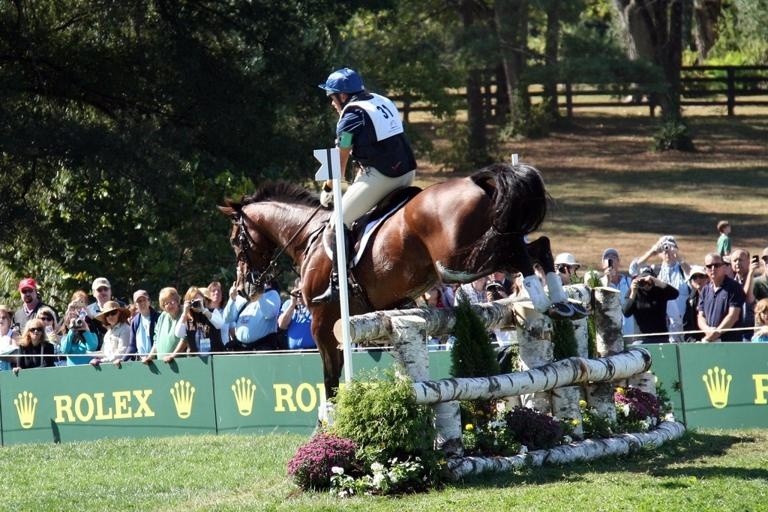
[319,284,686,482]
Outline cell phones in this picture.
[608,259,614,268]
[752,255,760,267]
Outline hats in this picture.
[92,278,111,290]
[133,289,149,302]
[96,301,131,321]
[554,253,581,270]
[602,249,620,263]
[185,286,212,302]
[761,247,768,258]
[318,68,364,92]
[0,305,15,314]
[19,279,36,290]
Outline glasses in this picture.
[30,327,43,332]
[41,317,53,322]
[706,263,723,268]
[105,310,117,317]
[22,289,33,293]
[97,287,108,292]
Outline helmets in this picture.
[326,90,340,96]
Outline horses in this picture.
[216,164,554,401]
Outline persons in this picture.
[263,272,277,292]
[731,248,751,341]
[112,290,161,370]
[0,305,21,369]
[29,306,64,367]
[208,281,225,315]
[62,301,98,366]
[85,277,120,324]
[144,287,191,364]
[12,319,52,374]
[716,221,732,255]
[553,251,587,285]
[11,278,58,326]
[165,286,223,363]
[752,247,768,299]
[628,235,694,343]
[681,266,710,343]
[63,290,88,309]
[622,266,679,344]
[310,68,418,303]
[278,278,317,349]
[424,265,554,343]
[222,278,282,353]
[750,299,768,342]
[696,254,746,342]
[743,255,765,295]
[600,249,634,343]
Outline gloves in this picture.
[320,191,334,207]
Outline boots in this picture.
[312,227,353,303]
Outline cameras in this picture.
[70,318,84,329]
[555,263,570,274]
[490,288,504,300]
[659,241,675,253]
[186,299,201,312]
[634,277,649,287]
